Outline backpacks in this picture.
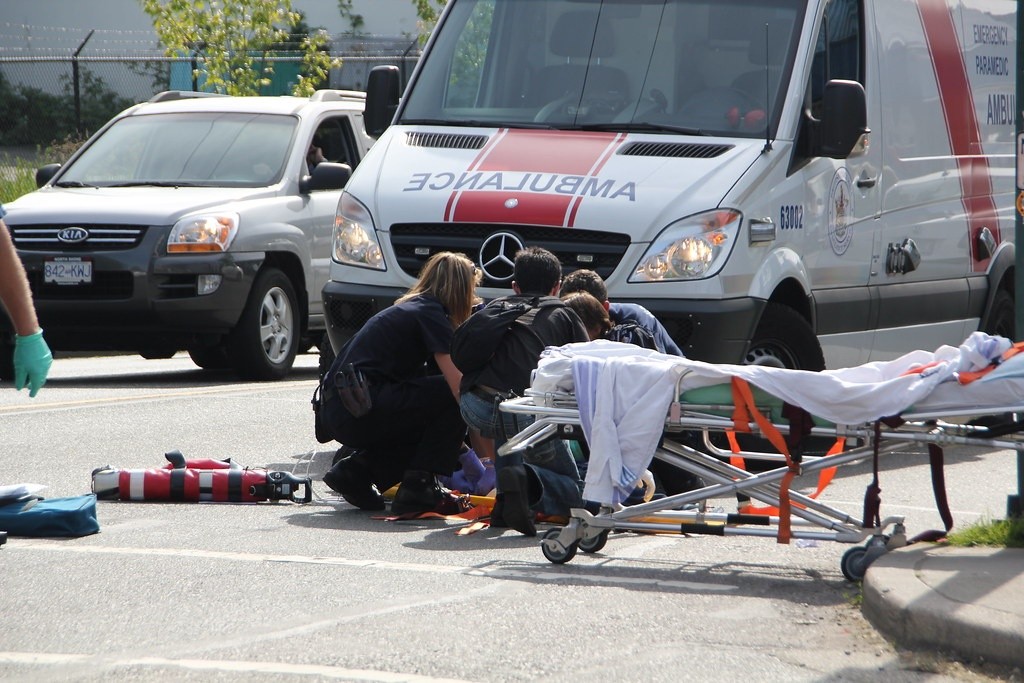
[450,293,568,376]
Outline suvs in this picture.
[1,89,405,383]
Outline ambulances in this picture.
[322,0,1024,465]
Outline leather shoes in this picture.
[490,466,538,536]
[322,450,386,511]
[391,470,473,515]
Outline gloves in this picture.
[452,448,496,496]
[637,468,656,503]
[13,327,53,397]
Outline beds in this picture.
[498,332,1024,580]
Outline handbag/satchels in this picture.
[311,382,336,443]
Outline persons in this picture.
[452,246,591,538]
[555,265,707,502]
[315,250,480,512]
[279,134,327,175]
[0,206,52,398]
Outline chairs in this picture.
[729,17,795,138]
[517,10,619,105]
